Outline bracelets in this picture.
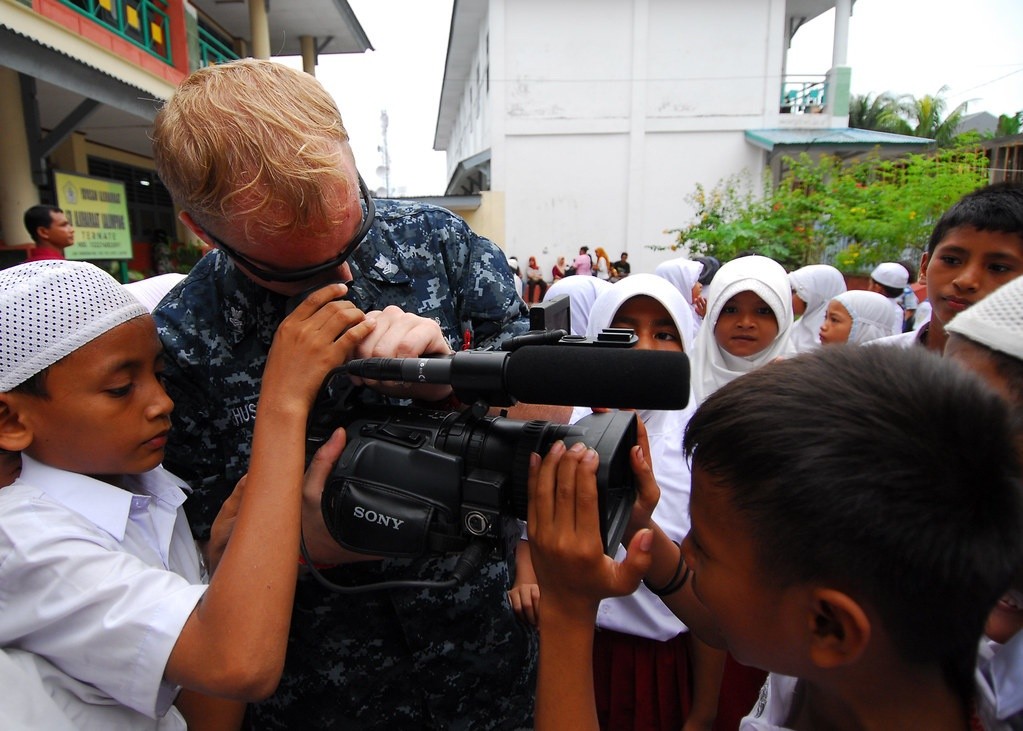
[654,567,691,596]
[643,539,684,594]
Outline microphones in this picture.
[344,344,690,411]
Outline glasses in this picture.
[199,166,375,285]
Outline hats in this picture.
[0,259,151,393]
[943,276,1023,359]
[870,263,910,289]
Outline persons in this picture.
[150,57,538,730]
[507,178,1023,730]
[22,205,76,263]
[0,257,373,730]
